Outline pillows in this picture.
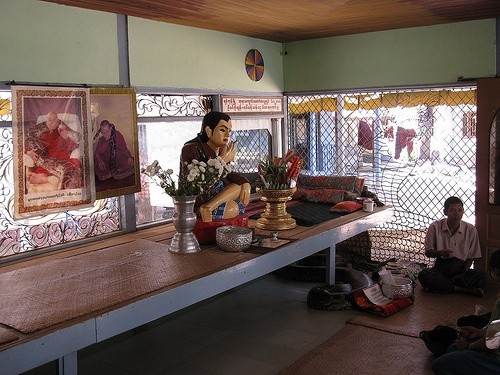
[330,201,362,212]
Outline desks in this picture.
[0,196,393,375]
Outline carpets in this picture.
[276,324,441,375]
[345,277,500,338]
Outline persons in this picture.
[431,249,500,375]
[180,111,251,222]
[418,197,485,297]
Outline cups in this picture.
[363,198,377,212]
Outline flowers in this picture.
[257,148,304,190]
[140,155,235,198]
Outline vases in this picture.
[255,186,298,230]
[168,197,201,254]
